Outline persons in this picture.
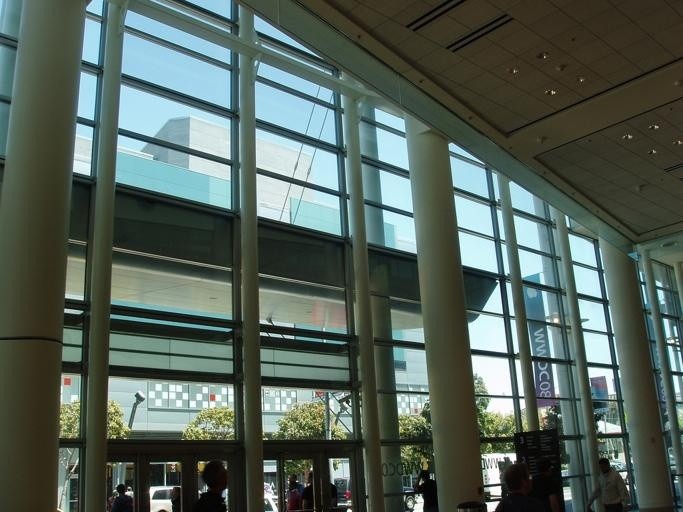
[299,471,314,511]
[170,486,182,512]
[327,475,338,508]
[285,480,302,511]
[528,457,565,512]
[586,457,631,512]
[495,462,548,512]
[287,472,306,496]
[413,469,440,512]
[106,484,134,512]
[188,459,230,512]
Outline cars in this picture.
[149,485,202,512]
[334,477,416,512]
